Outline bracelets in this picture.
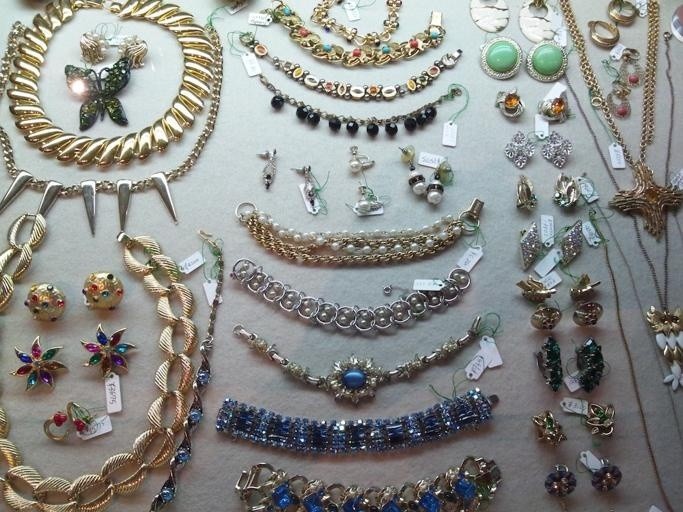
[231,0,466,140]
[230,456,502,509]
[221,257,474,335]
[212,396,492,456]
[227,195,488,266]
[223,313,489,403]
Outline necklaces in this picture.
[1,0,226,510]
[560,0,682,511]
